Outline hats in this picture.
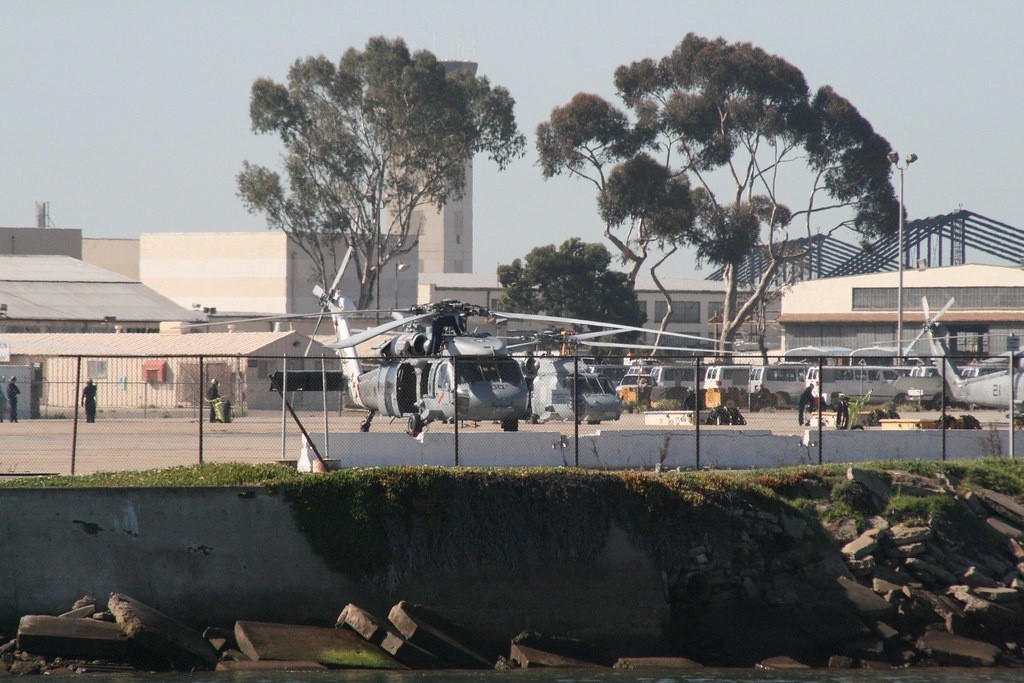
[687,386,693,393]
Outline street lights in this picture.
[709,310,725,365]
[886,151,918,364]
[393,259,411,310]
[201,305,217,396]
[743,313,758,342]
[363,192,394,327]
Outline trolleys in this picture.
[833,389,874,431]
[204,396,233,422]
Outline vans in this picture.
[593,346,973,411]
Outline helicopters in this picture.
[169,243,733,438]
[903,294,1024,429]
[390,308,738,426]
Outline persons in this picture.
[684,386,697,417]
[81,377,97,423]
[830,391,848,430]
[6,375,21,423]
[205,378,220,423]
[797,383,816,426]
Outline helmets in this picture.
[10,376,16,381]
[86,378,92,383]
[212,379,218,385]
